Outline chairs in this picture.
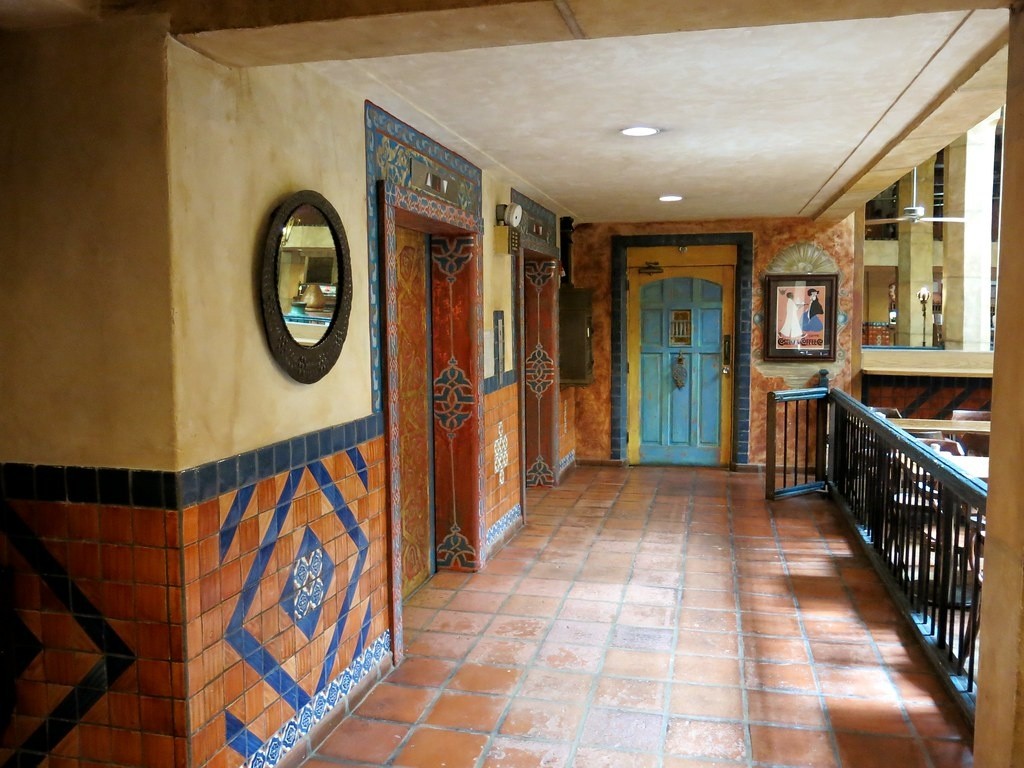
[869,404,986,668]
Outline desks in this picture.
[886,416,991,434]
[914,453,988,610]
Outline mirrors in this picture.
[262,190,354,384]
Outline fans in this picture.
[866,173,964,226]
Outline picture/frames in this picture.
[765,272,840,362]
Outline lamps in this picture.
[918,287,932,346]
